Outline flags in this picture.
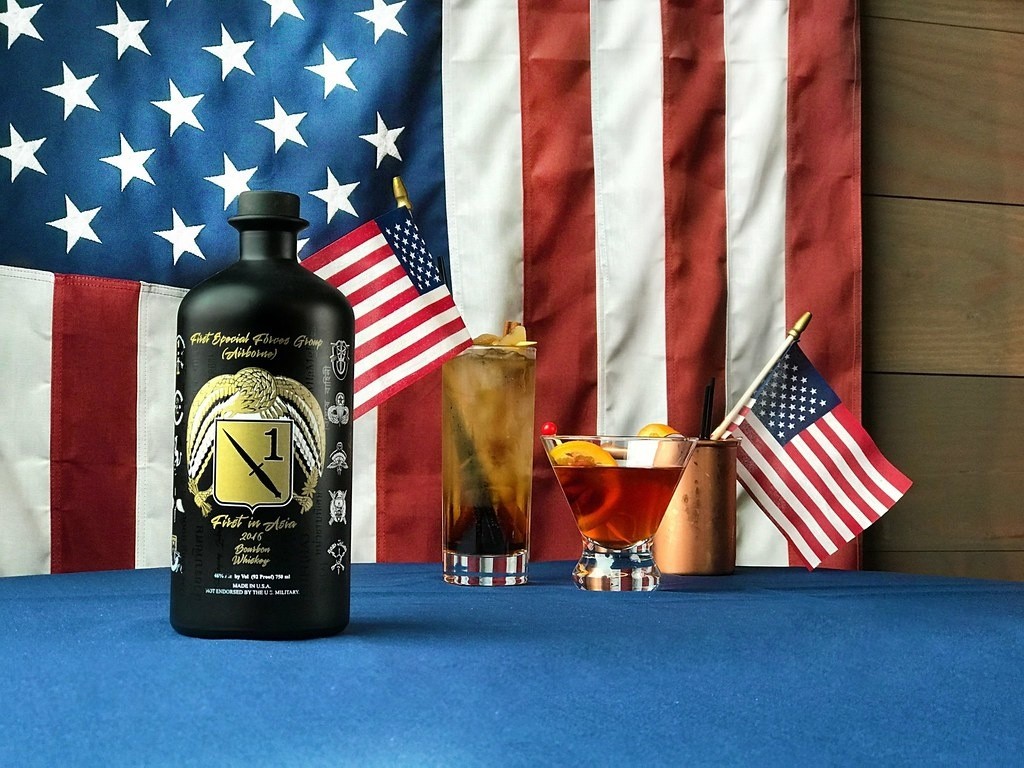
[0,0,865,575]
[718,338,913,572]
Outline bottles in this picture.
[173,190,355,640]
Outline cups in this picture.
[539,432,700,594]
[644,436,743,577]
[439,340,537,588]
